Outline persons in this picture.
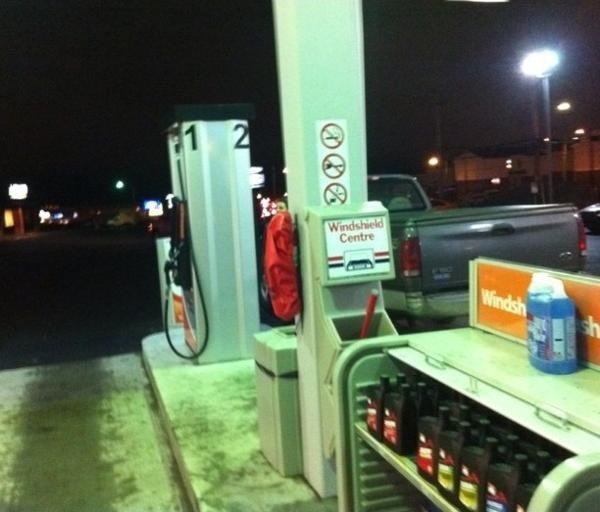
[274,198,287,214]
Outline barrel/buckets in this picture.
[526,272,578,375]
[366,372,562,512]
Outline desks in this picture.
[332,327,600,512]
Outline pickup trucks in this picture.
[366,173,587,324]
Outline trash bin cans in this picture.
[251,324,301,477]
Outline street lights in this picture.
[115,181,136,203]
[519,49,593,204]
